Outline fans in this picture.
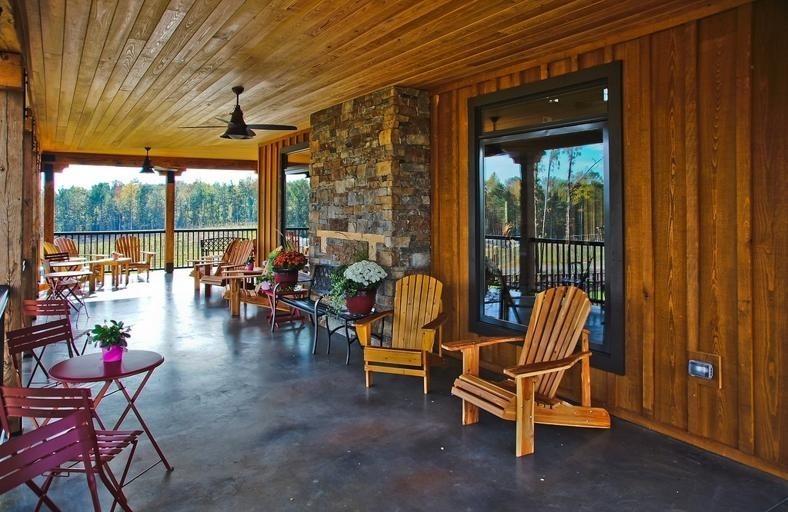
[178,86,298,131]
[139,146,178,174]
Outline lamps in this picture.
[219,127,256,139]
